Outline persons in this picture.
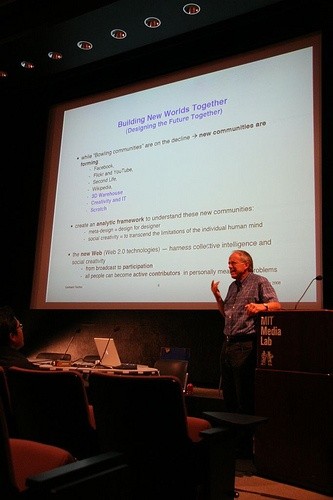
[211,250,281,459]
[0,309,49,371]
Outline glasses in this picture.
[9,324,23,333]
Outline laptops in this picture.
[93,337,138,368]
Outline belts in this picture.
[226,335,254,343]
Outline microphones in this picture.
[56,330,82,365]
[295,275,322,309]
[94,328,120,366]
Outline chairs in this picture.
[0,364,235,500]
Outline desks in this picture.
[28,359,159,375]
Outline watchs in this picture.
[263,303,269,312]
